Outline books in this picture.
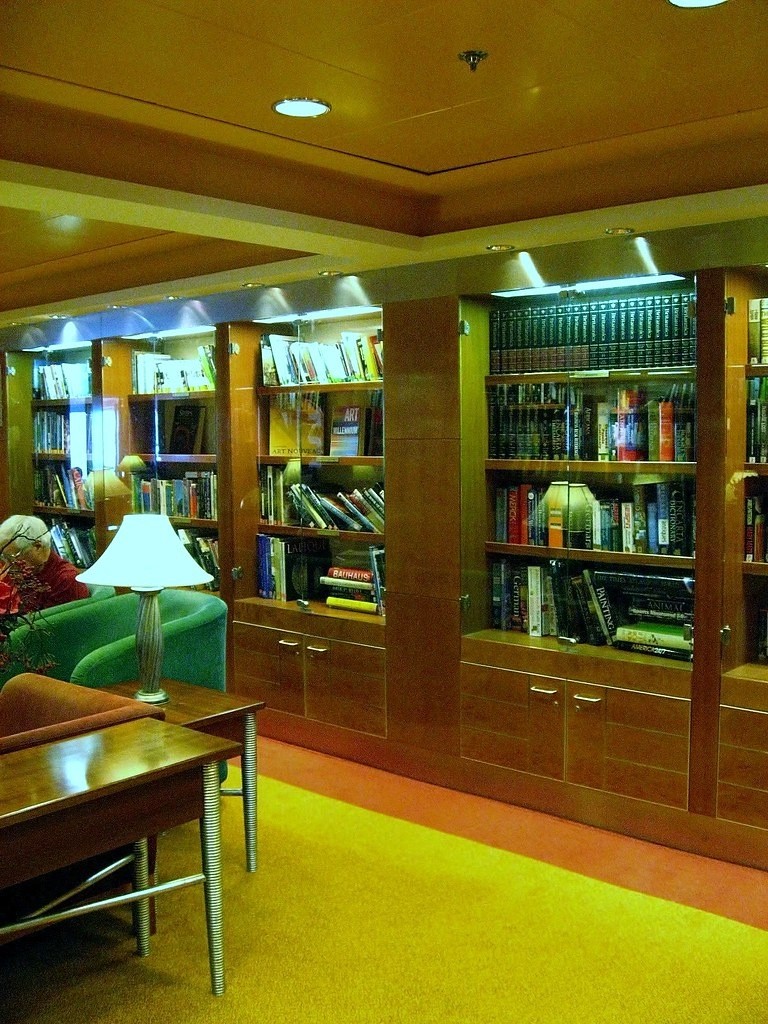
[25,274,767,677]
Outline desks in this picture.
[0,714,248,998]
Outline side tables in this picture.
[85,676,269,878]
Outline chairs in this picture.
[0,671,166,943]
[0,587,231,789]
[0,567,118,634]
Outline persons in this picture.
[67,466,89,503]
[0,514,88,617]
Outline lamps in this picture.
[73,511,217,709]
[522,477,620,650]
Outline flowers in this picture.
[0,551,65,676]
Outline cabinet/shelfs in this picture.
[1,260,768,873]
